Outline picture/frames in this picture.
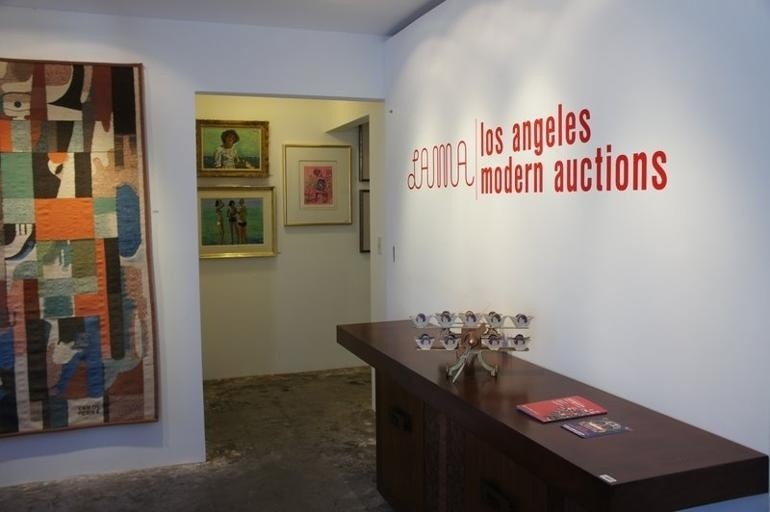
[0,57,160,438]
[197,119,270,177]
[359,125,372,182]
[358,188,369,253]
[198,184,278,259]
[283,145,351,225]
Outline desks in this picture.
[335,319,769,512]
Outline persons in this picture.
[213,129,253,169]
[237,199,249,244]
[214,198,225,244]
[226,200,240,243]
